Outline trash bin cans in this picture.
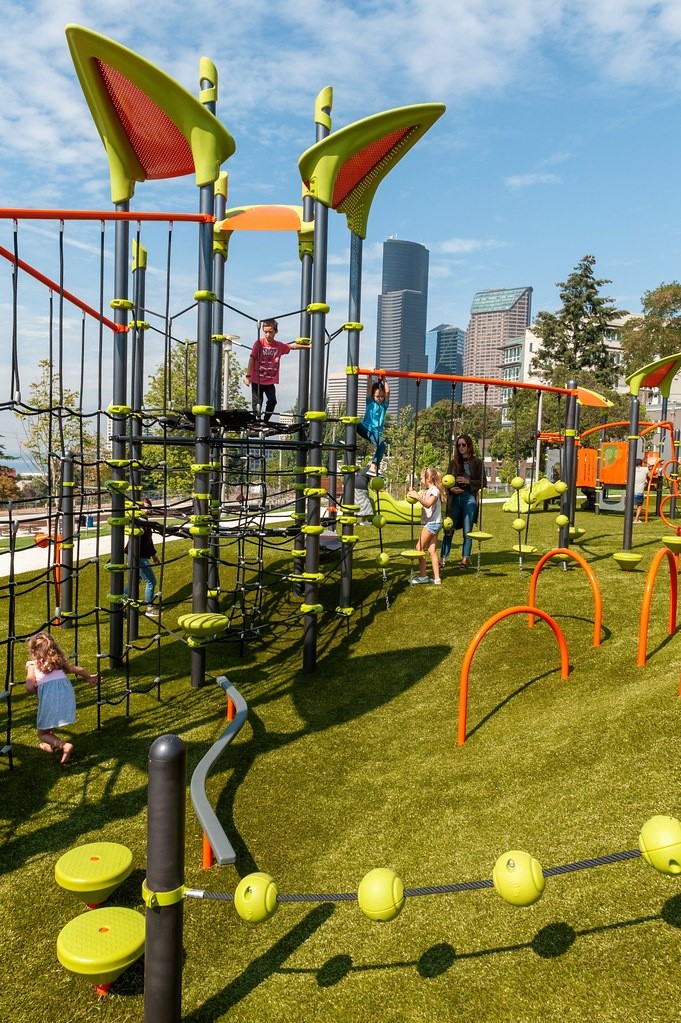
[86,516,93,527]
[80,515,86,526]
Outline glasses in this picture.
[457,443,467,447]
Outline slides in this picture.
[502,477,561,513]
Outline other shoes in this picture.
[366,469,376,476]
[429,577,442,585]
[439,563,445,570]
[408,575,428,584]
[460,564,466,570]
[632,520,641,523]
[145,608,163,616]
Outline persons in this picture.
[633,459,651,523]
[24,631,103,764]
[431,435,488,571]
[356,368,390,476]
[407,467,444,585]
[124,496,164,617]
[245,319,312,426]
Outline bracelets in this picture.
[246,374,251,378]
